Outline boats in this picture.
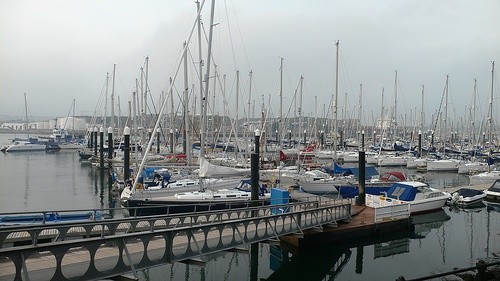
[5,1,499,215]
